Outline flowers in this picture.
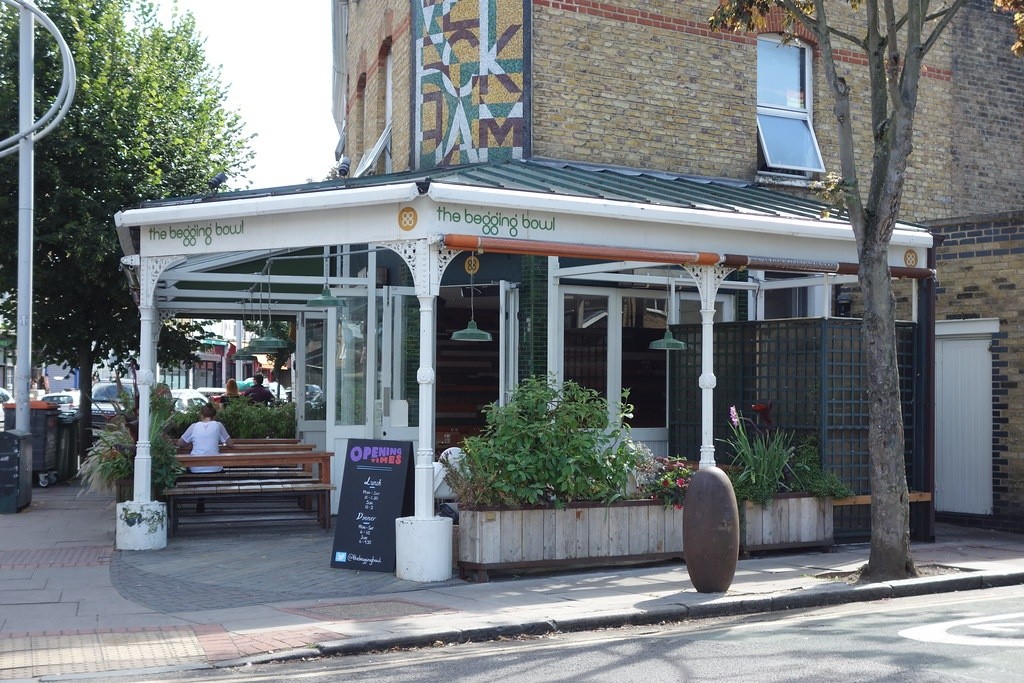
[444,373,830,503]
[66,382,174,492]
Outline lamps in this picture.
[236,291,254,355]
[209,170,226,193]
[450,250,492,342]
[230,311,258,360]
[338,157,351,178]
[837,294,850,305]
[248,270,286,348]
[648,266,687,350]
[306,259,345,308]
[238,277,277,354]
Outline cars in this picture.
[285,383,322,402]
[195,386,227,401]
[41,390,79,416]
[0,386,15,422]
[171,387,209,414]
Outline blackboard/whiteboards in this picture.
[329,438,415,572]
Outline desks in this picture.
[179,444,316,453]
[174,438,301,445]
[170,450,335,529]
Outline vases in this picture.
[116,478,161,501]
[456,490,835,580]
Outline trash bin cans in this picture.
[61,410,84,477]
[3,400,62,487]
[56,413,76,483]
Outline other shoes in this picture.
[197,504,205,512]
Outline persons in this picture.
[243,374,275,406]
[31,380,37,388]
[220,378,239,409]
[172,403,233,513]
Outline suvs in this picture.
[239,376,288,406]
[72,379,176,446]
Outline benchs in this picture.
[162,464,337,531]
